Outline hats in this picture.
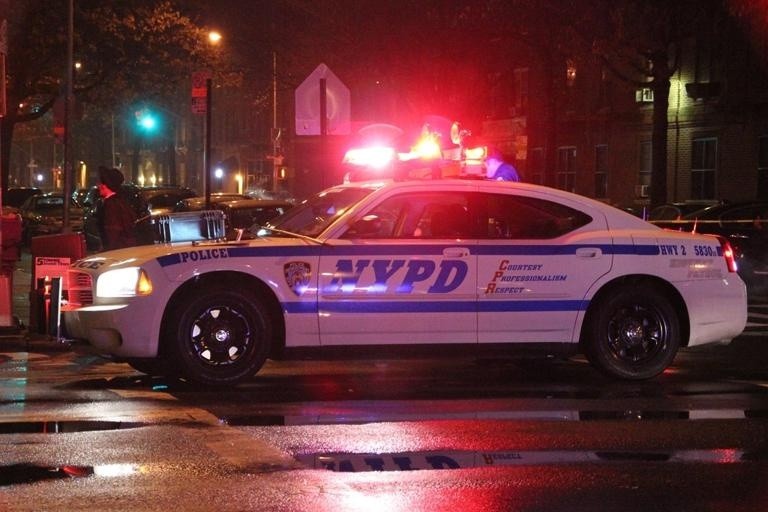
[99,166,125,192]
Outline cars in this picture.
[598,200,768,277]
[0,185,295,232]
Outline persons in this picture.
[97,181,134,249]
[480,147,520,181]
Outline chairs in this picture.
[427,203,470,238]
